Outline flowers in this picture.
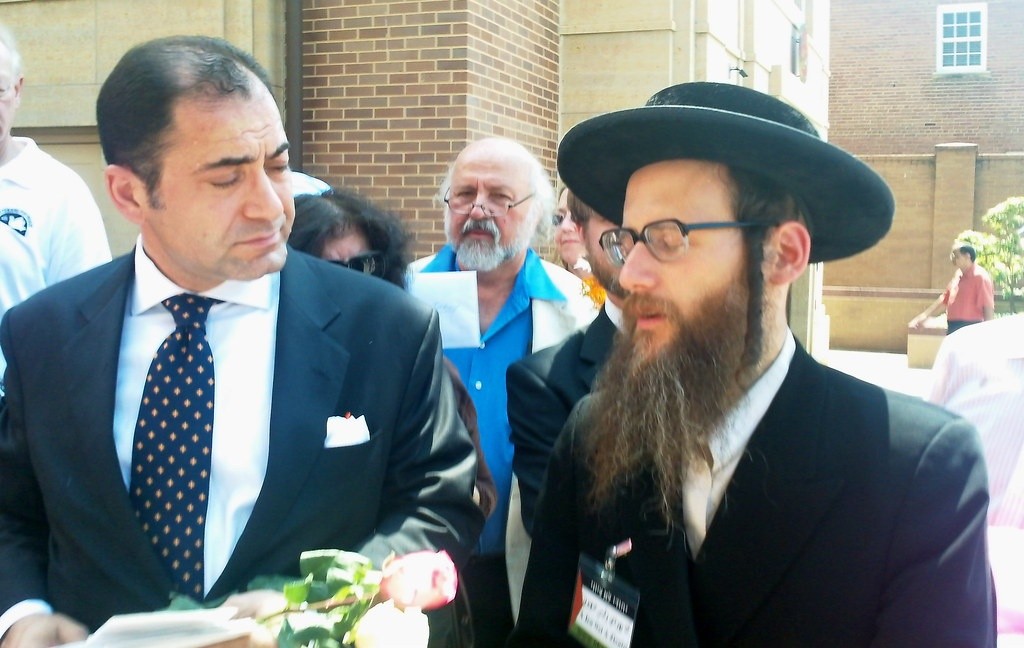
[251,549,458,648]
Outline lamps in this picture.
[729,68,748,77]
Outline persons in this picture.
[906,241,1024,648]
[517,84,997,648]
[0,31,630,648]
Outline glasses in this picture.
[598,219,772,267]
[552,213,574,226]
[444,185,533,217]
[326,250,386,280]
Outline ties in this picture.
[129,293,226,603]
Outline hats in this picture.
[556,81,895,264]
